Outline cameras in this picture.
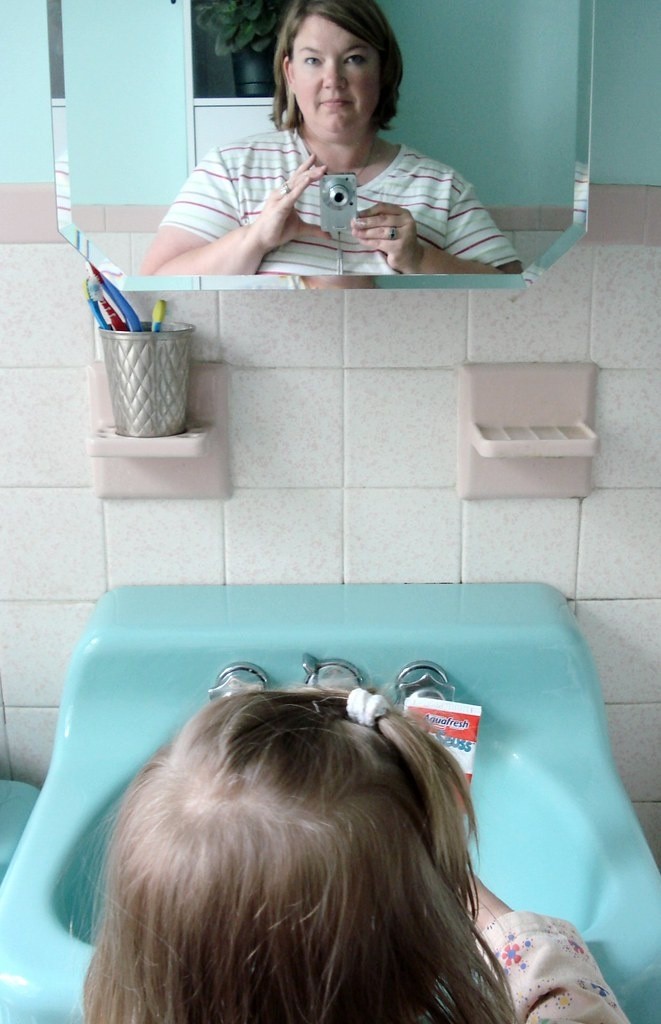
[319,173,357,232]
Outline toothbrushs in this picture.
[83,261,175,331]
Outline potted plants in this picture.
[194,0,286,97]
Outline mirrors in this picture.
[45,0,597,290]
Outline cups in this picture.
[98,322,196,438]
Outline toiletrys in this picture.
[401,696,483,786]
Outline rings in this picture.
[389,228,396,240]
[283,183,290,193]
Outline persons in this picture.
[136,1,525,273]
[78,686,629,1024]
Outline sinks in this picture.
[3,581,660,1022]
